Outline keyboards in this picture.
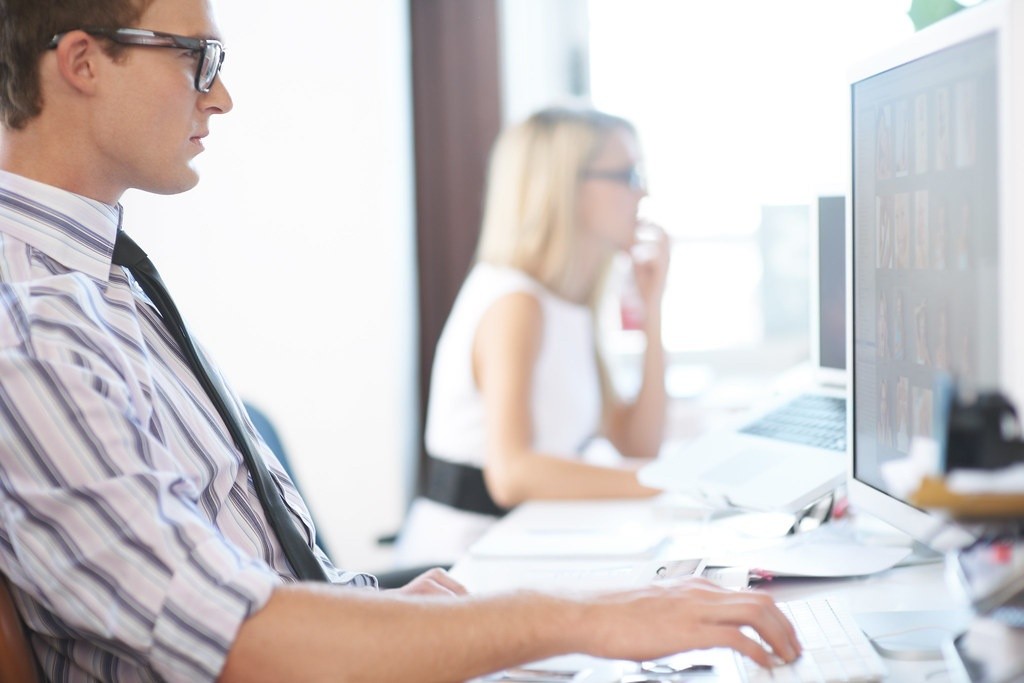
[716,596,888,683]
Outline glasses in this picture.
[576,160,648,193]
[47,25,226,92]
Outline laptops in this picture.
[696,189,848,513]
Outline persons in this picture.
[0,0,802,683]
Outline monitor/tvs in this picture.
[846,0,1024,660]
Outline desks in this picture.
[446,397,972,683]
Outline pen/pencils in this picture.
[749,568,776,583]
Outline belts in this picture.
[422,453,507,516]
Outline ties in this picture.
[112,229,326,585]
